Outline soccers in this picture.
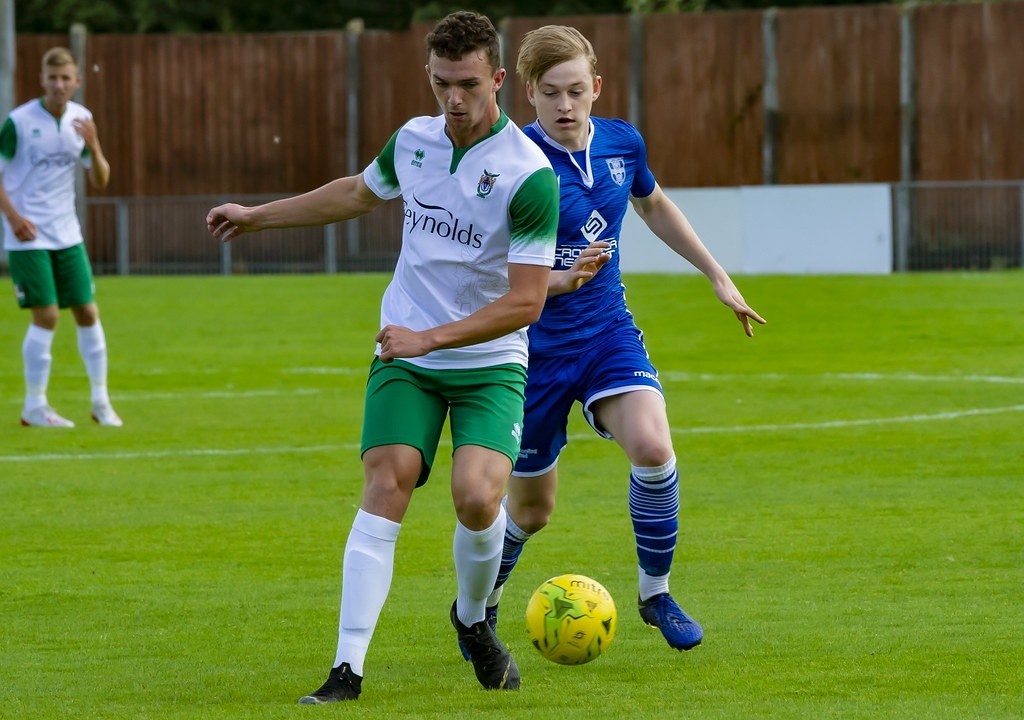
[523,572,618,666]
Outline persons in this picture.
[449,26,766,662]
[0,47,123,428]
[207,12,559,706]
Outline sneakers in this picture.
[19,405,76,430]
[638,591,704,650]
[299,662,364,705]
[460,603,498,660]
[450,598,519,693]
[90,403,123,425]
